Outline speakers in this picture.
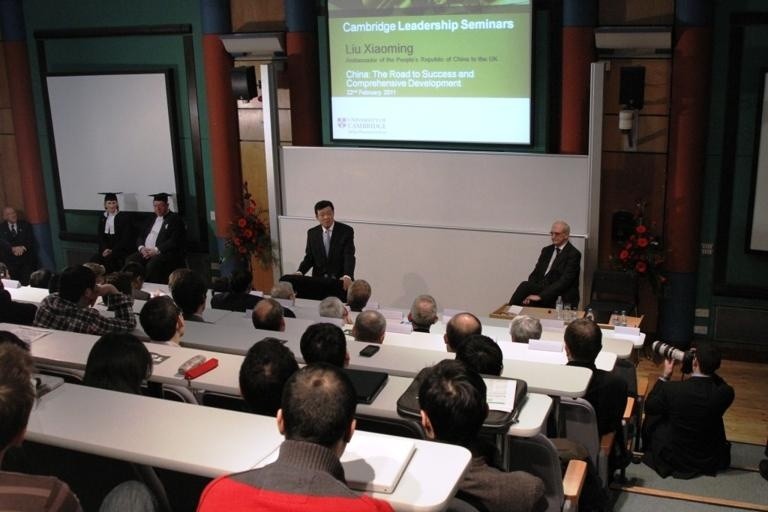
[230,66,258,100]
[618,66,646,106]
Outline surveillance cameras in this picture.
[619,110,632,134]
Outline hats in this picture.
[148,192,172,201]
[98,192,121,200]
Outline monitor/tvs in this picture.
[324,0,536,147]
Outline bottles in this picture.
[586,308,594,320]
[555,297,562,320]
[571,307,578,320]
[612,310,619,322]
[621,311,627,324]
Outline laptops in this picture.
[343,368,389,406]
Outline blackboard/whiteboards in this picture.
[276,216,589,312]
[278,145,590,237]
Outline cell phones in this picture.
[359,345,380,357]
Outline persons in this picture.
[509,220,581,310]
[210,270,297,318]
[31,263,137,334]
[418,359,547,512]
[510,311,544,347]
[318,296,352,324]
[295,199,356,290]
[407,292,439,334]
[252,298,285,331]
[0,205,45,284]
[641,340,735,479]
[169,269,211,322]
[352,310,386,345]
[82,332,152,393]
[98,271,134,308]
[125,193,188,266]
[0,341,157,512]
[271,280,296,306]
[347,279,372,312]
[240,336,299,415]
[29,267,52,288]
[563,317,628,434]
[82,262,105,285]
[443,312,482,352]
[88,192,136,274]
[122,263,150,300]
[299,323,348,367]
[195,365,394,512]
[458,335,503,376]
[138,295,187,346]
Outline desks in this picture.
[0,319,552,470]
[487,302,644,330]
[24,382,472,512]
[2,282,645,439]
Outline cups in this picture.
[565,304,571,321]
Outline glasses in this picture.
[550,232,565,236]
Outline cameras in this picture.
[651,341,693,374]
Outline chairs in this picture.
[558,392,639,505]
[511,433,588,511]
[582,269,640,324]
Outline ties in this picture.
[324,230,331,258]
[552,247,560,264]
[11,224,16,237]
